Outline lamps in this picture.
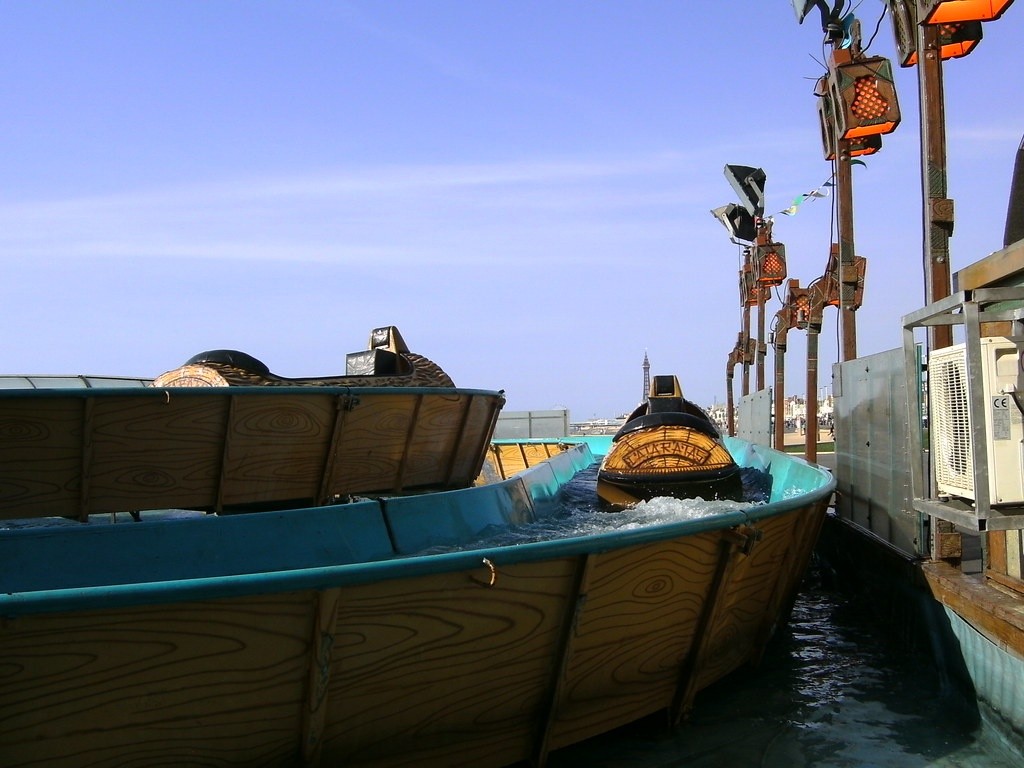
[709,162,765,246]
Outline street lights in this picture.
[793,0,901,364]
[789,278,841,461]
[726,332,757,439]
[710,203,772,396]
[725,164,786,393]
[887,0,1017,352]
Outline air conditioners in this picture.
[929,335,1024,507]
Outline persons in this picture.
[788,422,790,430]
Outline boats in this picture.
[596,376,744,514]
